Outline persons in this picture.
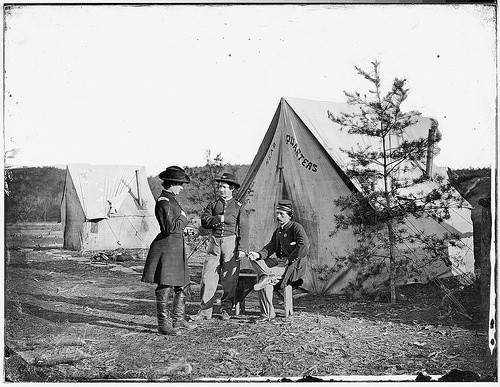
[140,165,197,335]
[246,201,310,323]
[188,173,249,320]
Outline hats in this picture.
[215,173,241,189]
[159,166,190,183]
[276,200,294,213]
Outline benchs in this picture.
[235,274,294,313]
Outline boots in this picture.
[155,285,181,336]
[171,285,197,329]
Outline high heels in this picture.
[188,307,213,320]
[221,308,231,321]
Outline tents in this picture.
[235,97,476,295]
[57,164,161,252]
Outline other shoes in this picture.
[254,274,276,291]
[254,316,269,323]
